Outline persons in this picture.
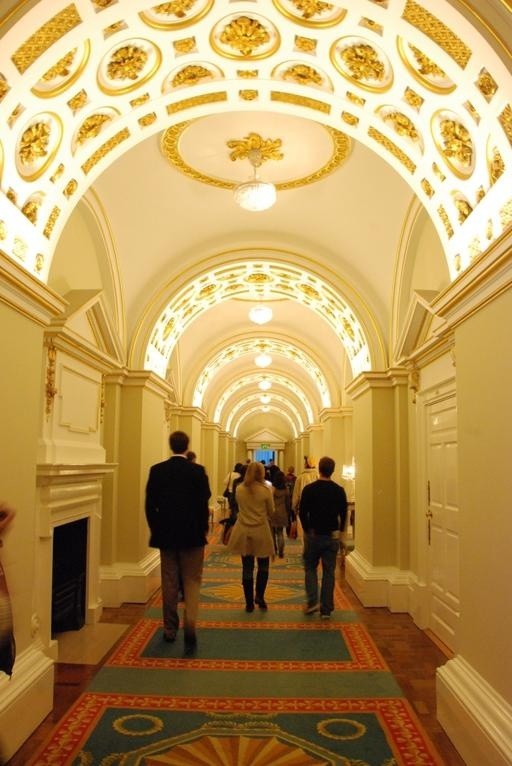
[226,462,275,612]
[298,457,347,619]
[178,451,214,600]
[224,458,297,559]
[144,431,211,654]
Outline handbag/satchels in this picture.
[290,521,297,539]
[224,488,230,497]
[223,518,237,544]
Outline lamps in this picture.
[234,149,276,212]
[249,306,273,414]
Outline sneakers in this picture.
[305,604,318,614]
[182,625,196,648]
[255,597,268,611]
[320,613,331,619]
[162,630,176,642]
[246,604,254,612]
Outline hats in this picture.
[304,456,316,467]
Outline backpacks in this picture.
[287,477,296,495]
